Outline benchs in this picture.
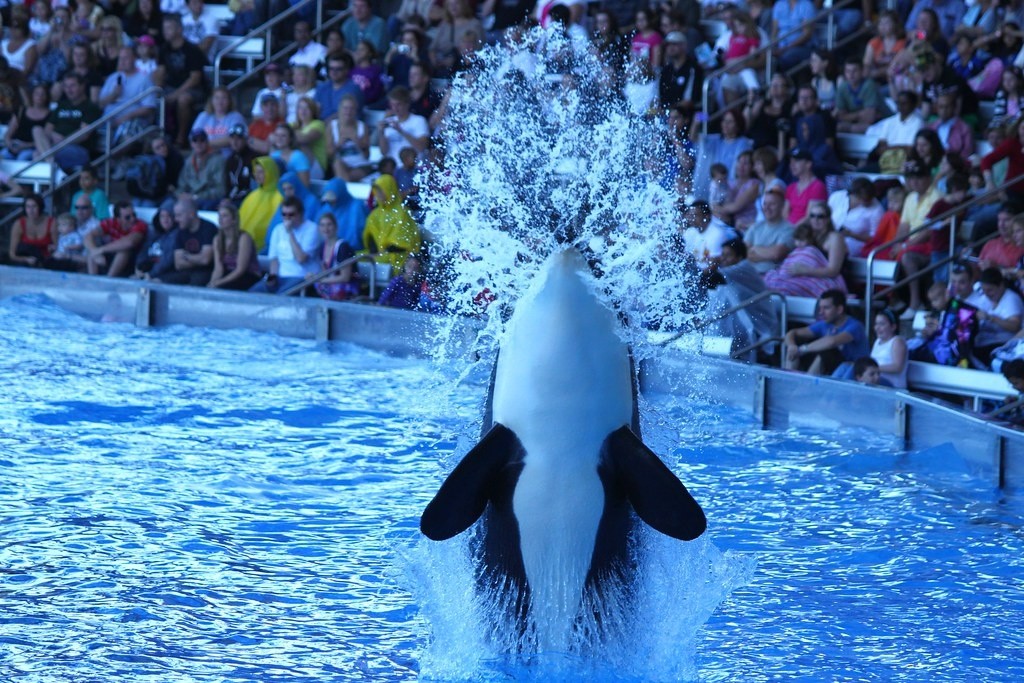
[0,21,1024,429]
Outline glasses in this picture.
[952,264,966,271]
[809,214,824,218]
[75,206,88,209]
[328,65,346,71]
[282,212,294,217]
[192,138,205,142]
[125,213,136,220]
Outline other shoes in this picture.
[899,303,926,321]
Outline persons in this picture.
[0,0,1024,427]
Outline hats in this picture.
[188,128,207,139]
[665,31,687,43]
[228,123,248,139]
[788,149,813,160]
[915,51,938,71]
[134,35,156,46]
